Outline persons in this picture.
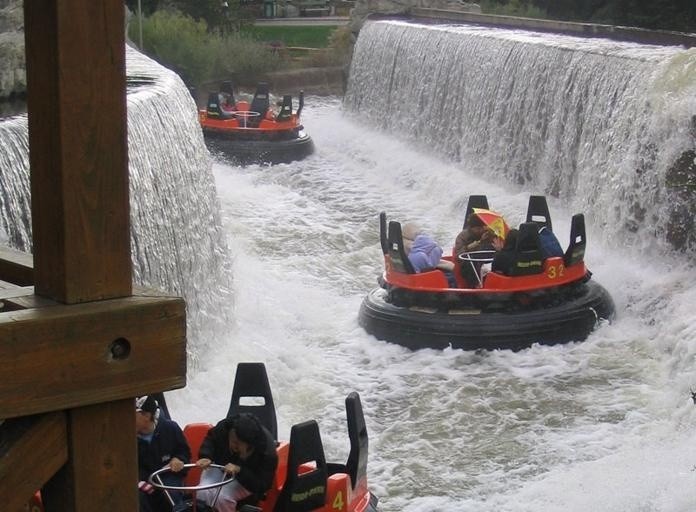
[194,412,276,512]
[135,396,192,512]
[401,207,565,289]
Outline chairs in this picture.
[122,363,372,512]
[204,79,306,130]
[378,191,587,290]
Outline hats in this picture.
[136,396,157,414]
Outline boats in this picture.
[187,79,316,166]
[357,192,618,351]
[137,359,384,512]
